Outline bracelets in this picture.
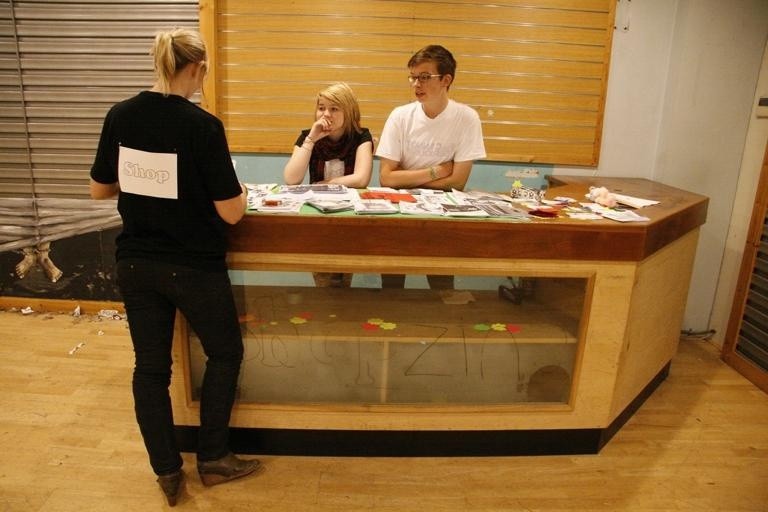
[430,164,438,181]
[306,133,317,145]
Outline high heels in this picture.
[197,453,259,487]
[158,469,187,507]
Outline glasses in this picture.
[407,72,444,86]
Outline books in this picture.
[238,181,532,220]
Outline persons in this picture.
[283,82,374,288]
[89,28,261,507]
[374,45,486,289]
[15,239,63,284]
